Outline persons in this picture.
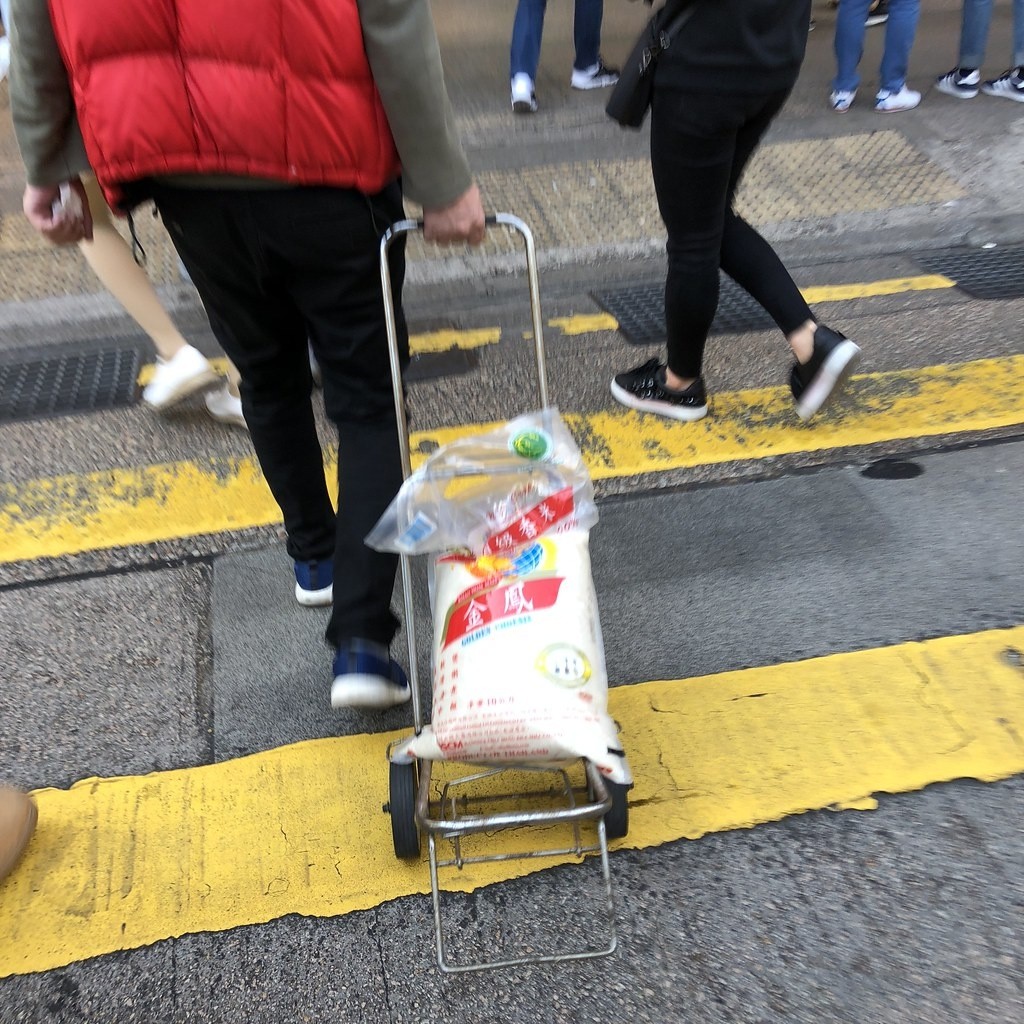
[605,0,863,423]
[509,0,620,112]
[828,0,921,114]
[932,0,1024,102]
[6,0,487,710]
[59,170,249,431]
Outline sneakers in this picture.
[328,645,412,708]
[608,358,710,421]
[205,386,252,431]
[142,341,224,408]
[292,552,332,607]
[829,85,854,111]
[787,324,861,421]
[874,86,920,115]
[508,73,538,115]
[572,58,621,92]
[981,67,1024,103]
[934,66,981,98]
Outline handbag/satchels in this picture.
[606,11,668,130]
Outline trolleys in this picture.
[382,214,632,973]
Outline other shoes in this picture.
[866,4,889,27]
[808,18,817,32]
[0,782,36,885]
